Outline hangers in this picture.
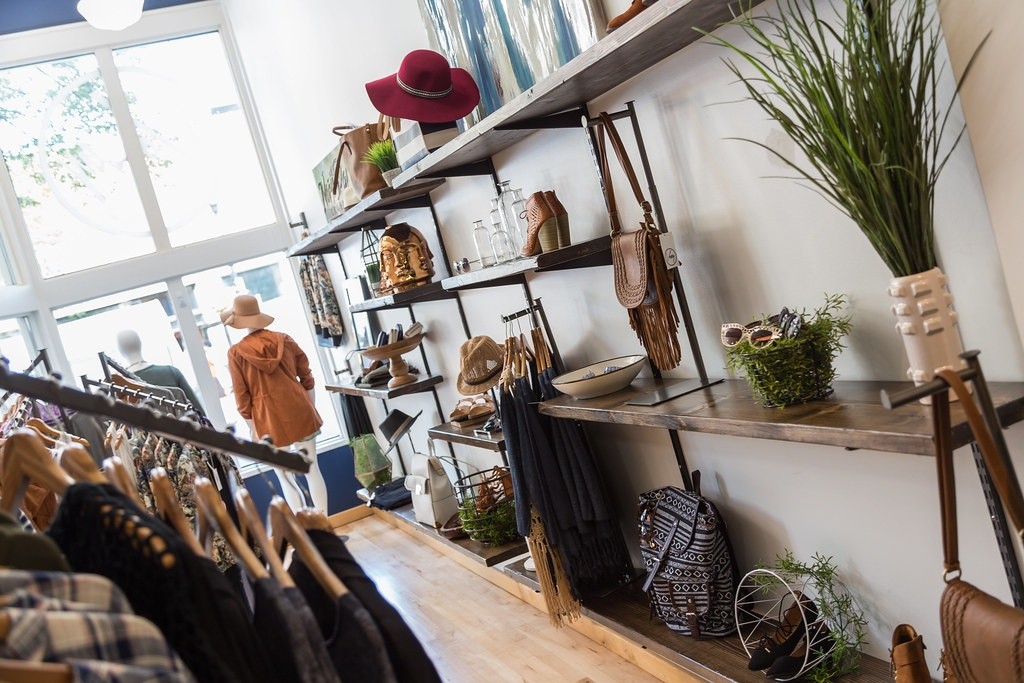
[0,371,348,683]
[300,227,308,242]
[498,302,552,394]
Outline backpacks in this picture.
[636,469,747,641]
[404,453,458,530]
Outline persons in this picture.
[220,295,328,516]
[379,222,436,294]
[115,329,206,417]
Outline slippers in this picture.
[449,398,473,421]
[468,394,499,418]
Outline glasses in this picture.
[720,322,783,349]
[779,307,802,339]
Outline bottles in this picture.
[497,180,521,257]
[473,220,497,269]
[490,222,516,264]
[511,188,528,245]
[490,197,505,231]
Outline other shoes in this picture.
[889,624,930,683]
[606,0,659,34]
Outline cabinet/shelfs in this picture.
[287,179,459,563]
[391,0,1024,683]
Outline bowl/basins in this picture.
[550,354,647,400]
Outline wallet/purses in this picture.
[376,321,423,347]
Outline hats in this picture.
[379,409,423,456]
[365,49,480,123]
[220,295,274,329]
[457,336,508,395]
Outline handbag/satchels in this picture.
[931,368,1024,683]
[596,112,682,370]
[312,126,398,224]
[376,111,461,172]
[367,475,412,510]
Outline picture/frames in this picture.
[341,275,375,371]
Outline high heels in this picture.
[748,591,835,679]
[519,191,558,256]
[544,189,571,247]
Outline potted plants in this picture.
[724,293,852,409]
[691,0,993,403]
